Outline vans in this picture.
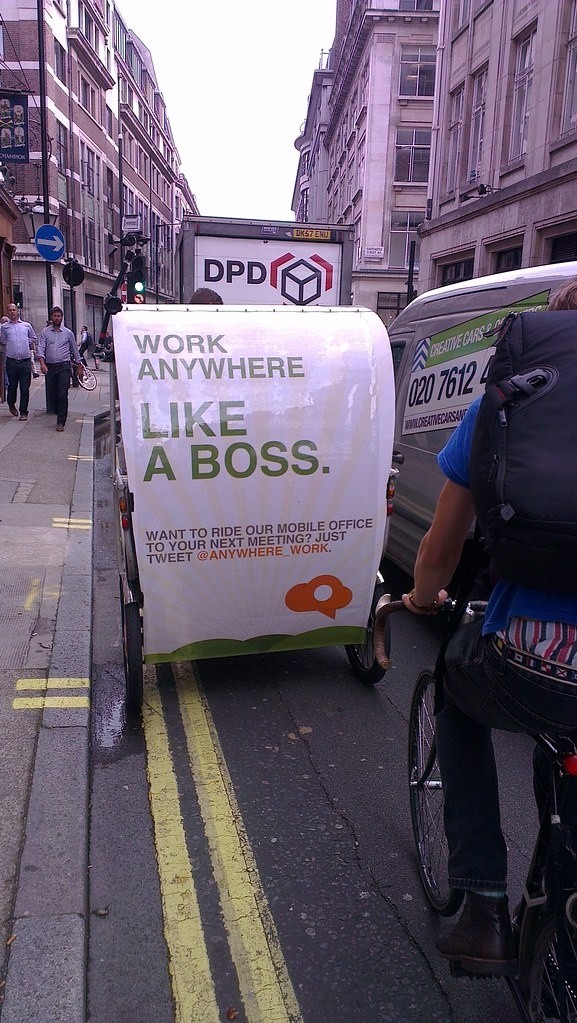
[382,257,577,593]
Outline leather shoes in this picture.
[434,890,517,974]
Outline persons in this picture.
[188,287,224,304]
[0,303,89,433]
[402,277,577,961]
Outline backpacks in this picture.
[470,309,577,561]
[86,332,93,345]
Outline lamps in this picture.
[459,194,482,203]
[477,184,504,195]
[0,161,31,215]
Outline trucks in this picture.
[174,210,356,306]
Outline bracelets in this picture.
[408,590,437,610]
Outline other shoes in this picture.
[9,406,18,416]
[33,373,40,378]
[56,424,64,433]
[20,412,28,421]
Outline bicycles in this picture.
[372,593,577,1023]
[67,351,98,391]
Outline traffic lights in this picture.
[126,255,147,304]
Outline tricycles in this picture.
[108,299,399,710]
